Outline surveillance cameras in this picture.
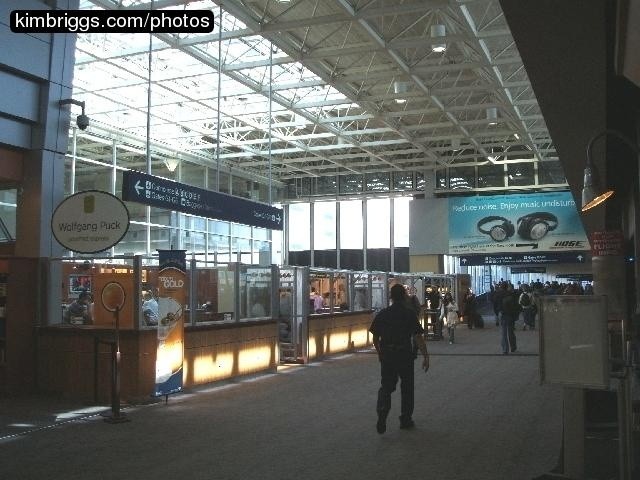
[77,114,89,130]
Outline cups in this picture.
[158,297,183,341]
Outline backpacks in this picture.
[520,292,530,306]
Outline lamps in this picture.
[485,107,498,125]
[430,11,445,53]
[579,129,640,211]
[393,69,407,103]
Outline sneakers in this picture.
[377,415,386,434]
[400,421,414,429]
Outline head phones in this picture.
[516,212,558,240]
[477,214,515,242]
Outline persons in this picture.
[460,289,478,330]
[427,285,443,339]
[489,277,592,354]
[403,284,422,360]
[438,292,459,345]
[250,295,265,318]
[367,284,431,434]
[142,291,158,326]
[64,291,90,324]
[308,286,325,313]
